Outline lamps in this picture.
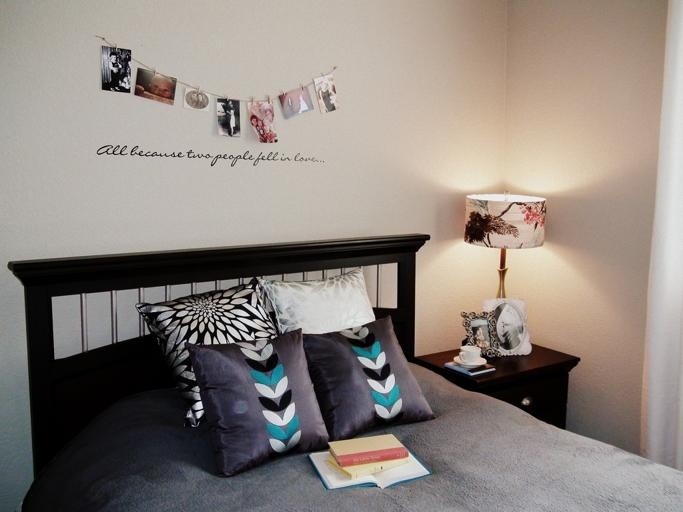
[464,194,546,298]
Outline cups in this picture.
[459,346,481,363]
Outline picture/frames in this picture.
[460,310,503,357]
[485,298,532,356]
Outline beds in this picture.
[7,234,683,511]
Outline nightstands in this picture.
[414,345,581,431]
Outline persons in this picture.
[250,109,277,142]
[109,52,122,91]
[319,83,336,112]
[226,105,235,136]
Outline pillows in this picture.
[255,266,377,335]
[303,314,437,441]
[186,328,330,476]
[135,277,282,430]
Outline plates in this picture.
[453,356,487,368]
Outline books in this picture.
[307,433,433,491]
[444,361,497,377]
